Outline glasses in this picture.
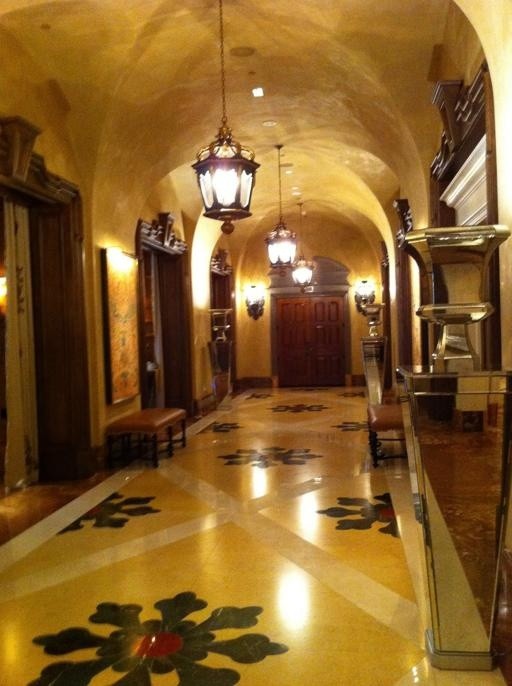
[353,275,377,311]
[246,283,266,322]
[264,144,317,293]
[191,1,262,234]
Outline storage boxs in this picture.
[103,407,189,468]
[367,402,406,470]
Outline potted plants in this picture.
[101,247,144,405]
